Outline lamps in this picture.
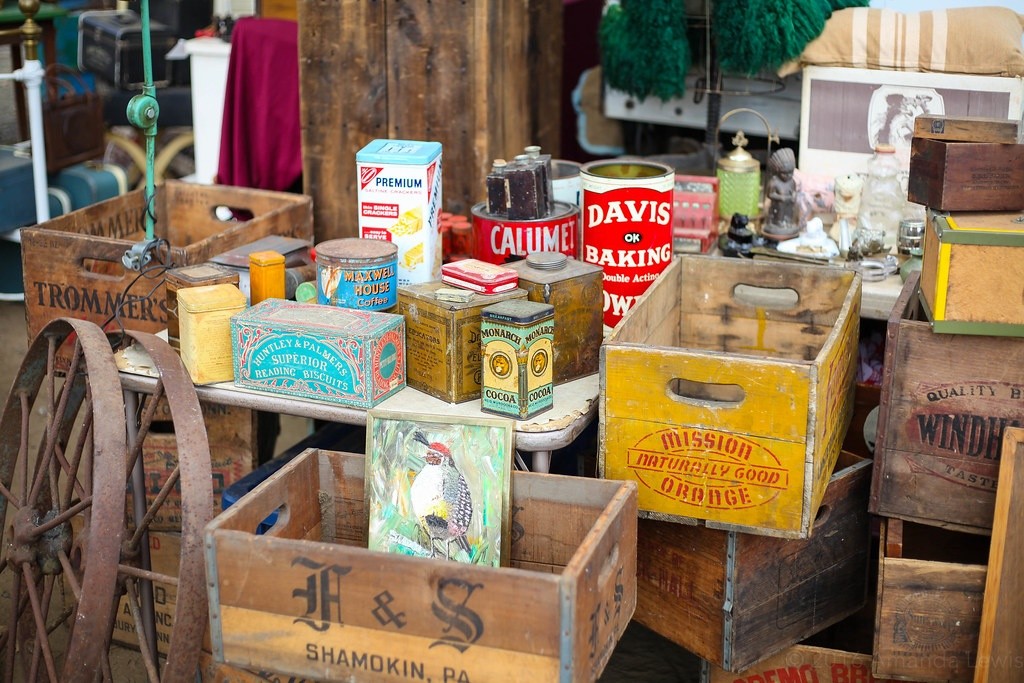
[712,106,773,238]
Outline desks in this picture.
[111,322,601,683]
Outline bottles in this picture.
[485,146,555,219]
[858,143,902,255]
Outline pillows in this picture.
[800,4,1024,78]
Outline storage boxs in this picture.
[871,519,989,683]
[229,297,408,412]
[907,136,1024,212]
[20,180,315,377]
[628,448,874,673]
[593,253,863,540]
[124,390,283,530]
[58,497,181,660]
[867,270,1024,537]
[397,280,531,405]
[482,300,556,422]
[500,252,604,388]
[919,206,1024,338]
[700,644,889,683]
[203,447,639,683]
